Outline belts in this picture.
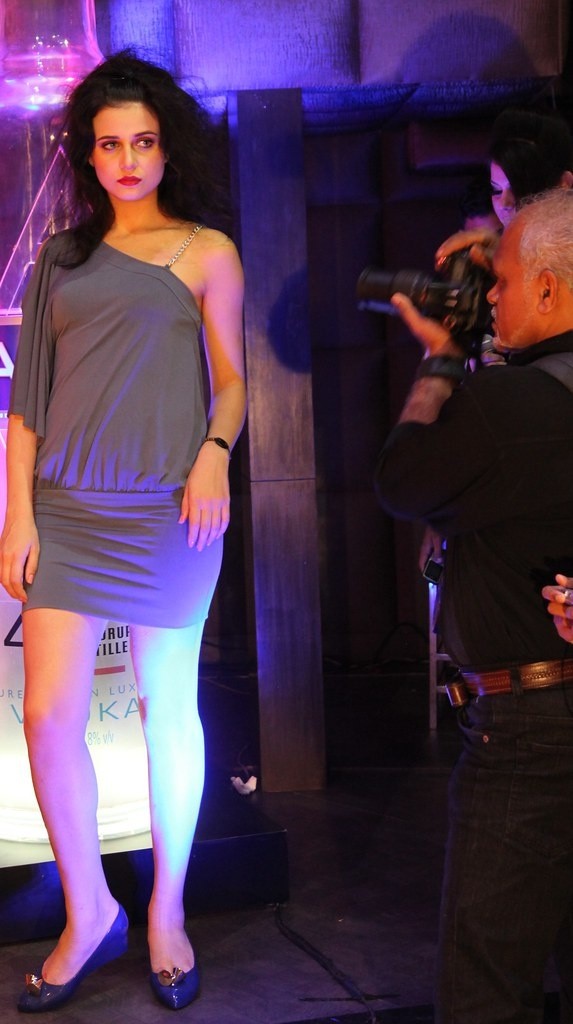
[445,658,573,708]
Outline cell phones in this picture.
[422,549,445,584]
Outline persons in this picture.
[377,108,573,1024]
[0,56,246,1011]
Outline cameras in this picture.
[357,247,495,357]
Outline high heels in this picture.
[18,904,128,1013]
[148,930,200,1010]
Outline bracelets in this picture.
[202,437,233,461]
